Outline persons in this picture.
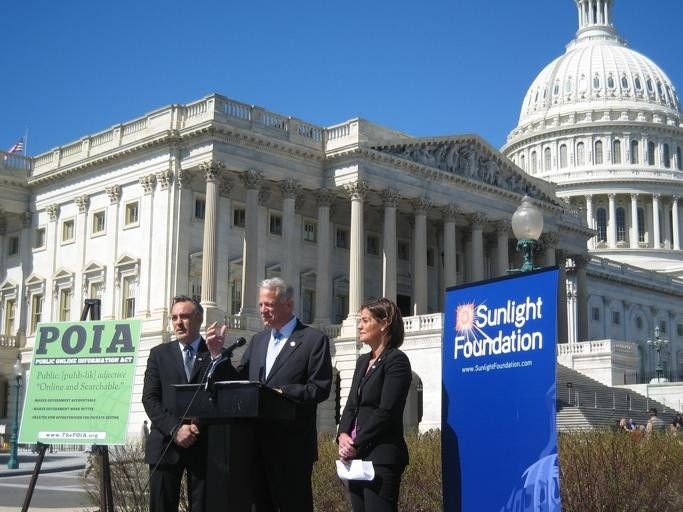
[142,295,229,512]
[619,408,683,435]
[335,299,412,512]
[205,276,332,511]
[142,420,150,451]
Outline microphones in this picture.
[212,337,248,356]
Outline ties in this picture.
[266,331,283,378]
[183,344,194,383]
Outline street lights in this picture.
[505,193,546,273]
[646,326,669,378]
[8,354,23,469]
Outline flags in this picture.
[4,136,23,160]
